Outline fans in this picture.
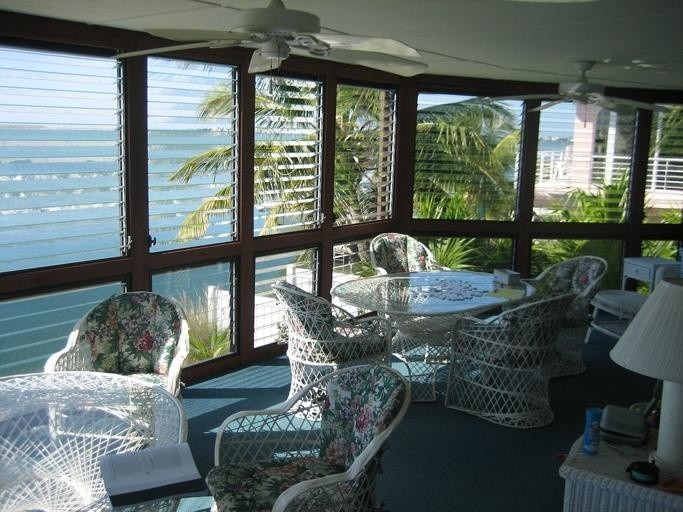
[111,0,428,78]
[493,61,671,116]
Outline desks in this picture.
[0,371,189,512]
[619,257,682,320]
[330,272,536,401]
[558,433,683,512]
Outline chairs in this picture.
[44,291,190,451]
[444,293,577,429]
[370,233,451,363]
[502,255,608,379]
[271,280,392,422]
[205,364,411,512]
[584,265,680,344]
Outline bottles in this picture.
[580,406,602,456]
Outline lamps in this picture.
[609,277,683,479]
